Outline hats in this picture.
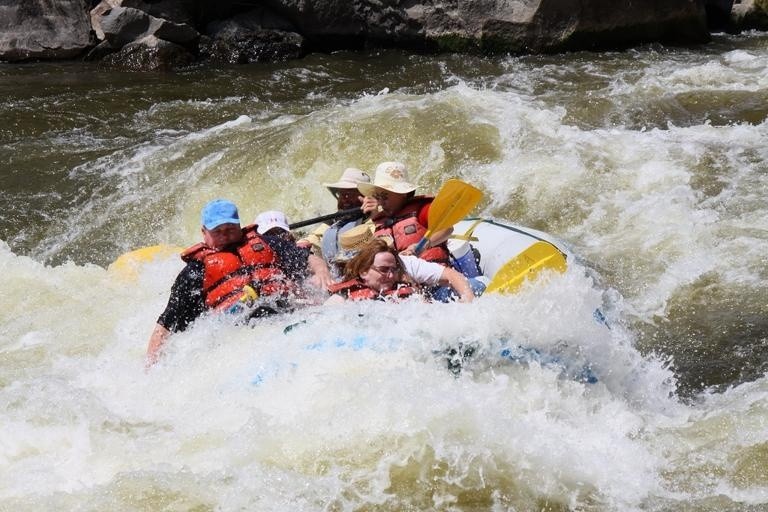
[254,211,290,235]
[331,224,394,263]
[325,162,420,197]
[201,199,240,231]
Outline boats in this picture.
[109,210,603,395]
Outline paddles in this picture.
[481,242,568,298]
[104,205,370,273]
[407,178,485,253]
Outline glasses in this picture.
[337,191,356,198]
[369,265,400,273]
[372,193,390,199]
